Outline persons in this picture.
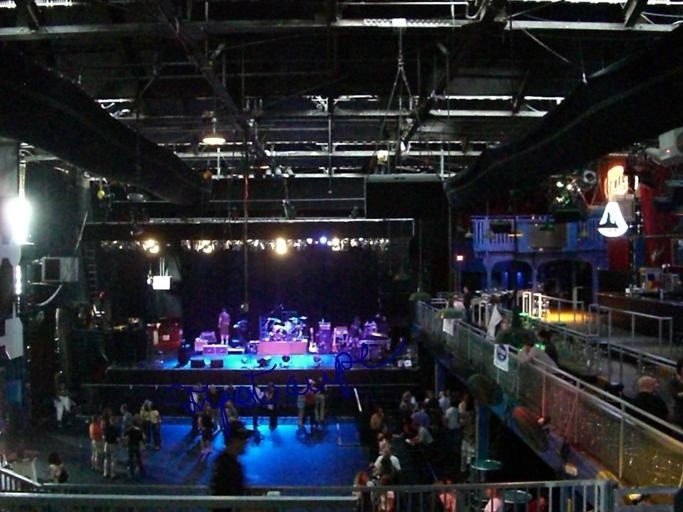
[118,403,135,440]
[210,421,259,511]
[102,423,121,481]
[139,399,153,451]
[188,382,242,457]
[51,362,73,431]
[380,314,390,335]
[87,413,105,474]
[47,454,70,486]
[216,305,230,344]
[535,492,549,511]
[539,329,559,366]
[673,358,683,419]
[517,342,559,379]
[627,375,670,430]
[122,418,146,482]
[462,284,473,314]
[296,379,333,435]
[453,294,463,309]
[239,316,248,337]
[264,382,280,430]
[483,485,505,512]
[356,386,477,512]
[146,401,163,452]
[249,383,265,433]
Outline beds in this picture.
[597,294,683,336]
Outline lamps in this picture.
[600,200,628,237]
